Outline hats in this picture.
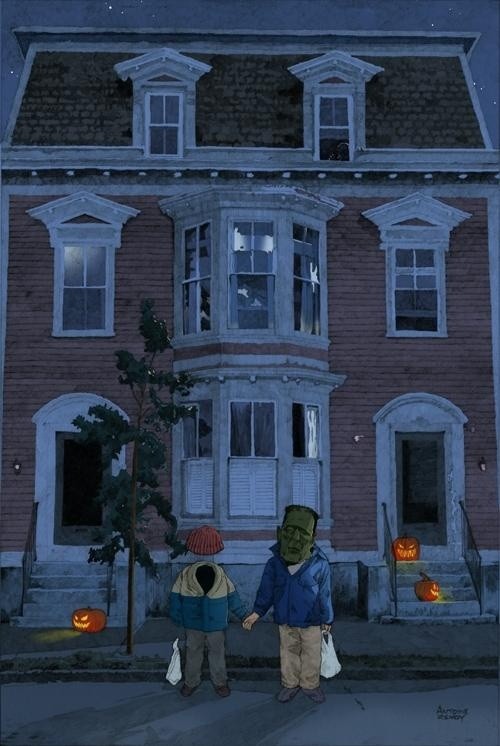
[186,525,226,557]
